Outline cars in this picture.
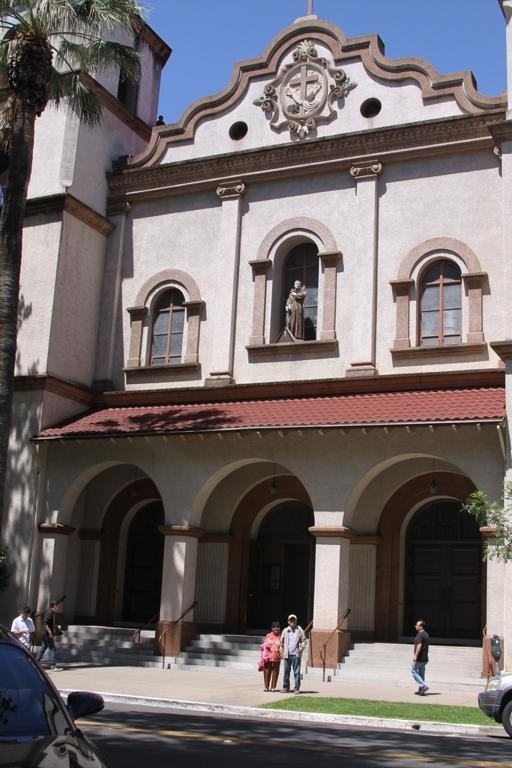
[478,668,511,747]
[0,620,116,767]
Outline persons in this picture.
[10,605,35,651]
[285,279,306,340]
[279,612,307,693]
[410,618,429,696]
[261,620,283,692]
[35,599,64,671]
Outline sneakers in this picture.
[414,685,429,696]
[264,687,301,694]
[51,666,64,672]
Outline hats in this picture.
[288,614,297,620]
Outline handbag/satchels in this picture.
[258,659,267,671]
